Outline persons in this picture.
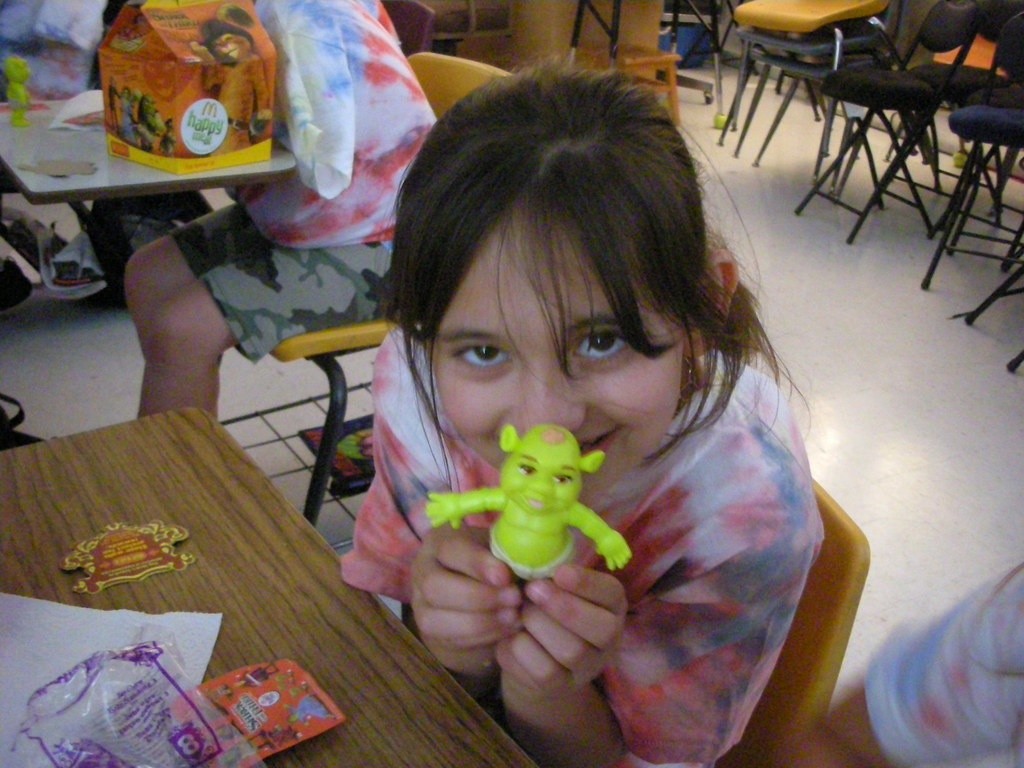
[0,0,440,427]
[769,561,1024,768]
[339,59,825,768]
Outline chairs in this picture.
[219,1,1024,550]
[714,475,871,767]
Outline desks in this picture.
[1,97,297,205]
[0,404,543,767]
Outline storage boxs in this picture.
[96,1,275,176]
